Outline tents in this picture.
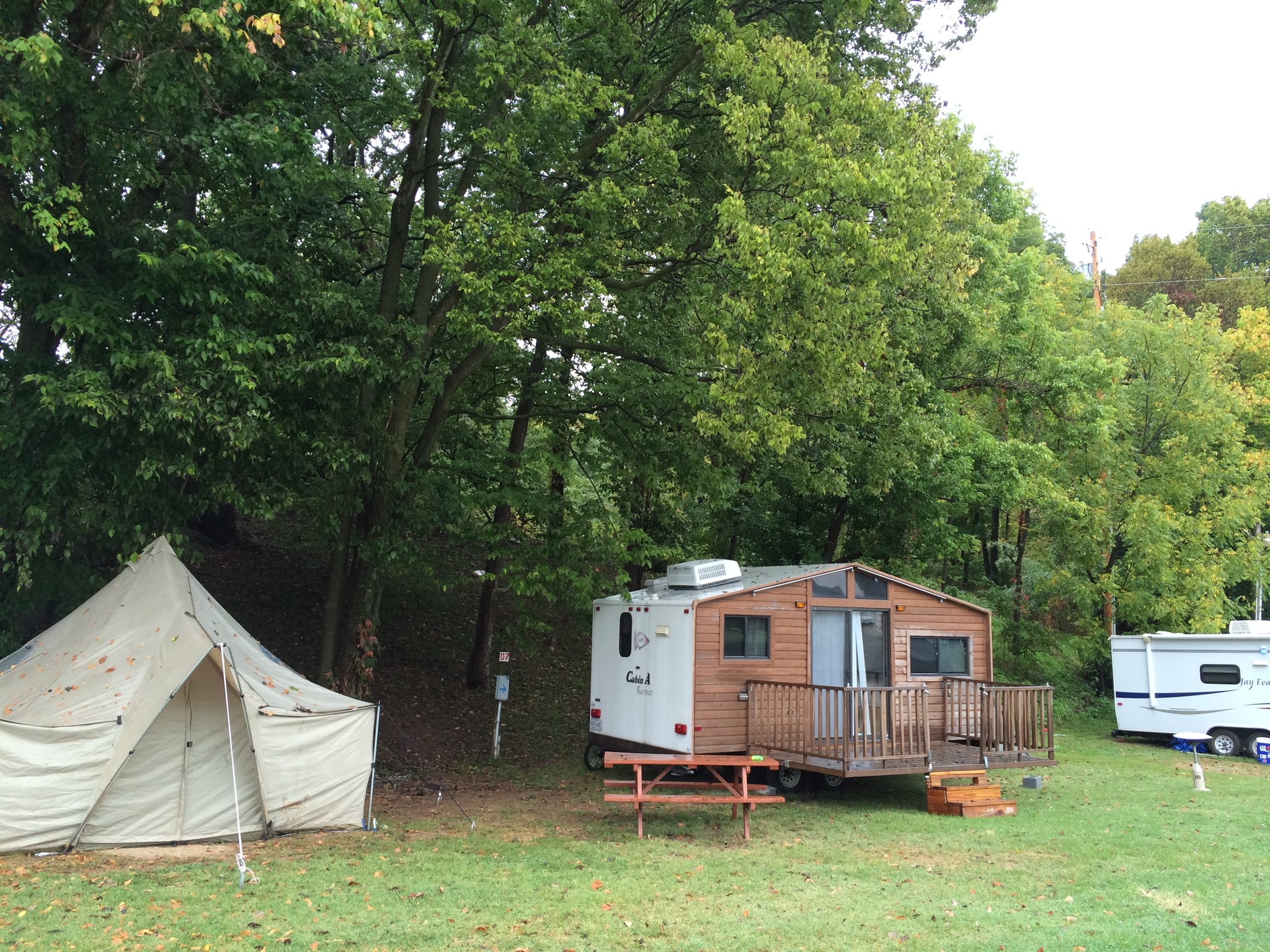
[0,536,380,856]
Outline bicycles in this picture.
[585,709,612,772]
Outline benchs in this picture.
[604,793,784,839]
[604,779,769,795]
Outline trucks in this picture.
[1107,620,1270,759]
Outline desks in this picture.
[603,753,779,839]
